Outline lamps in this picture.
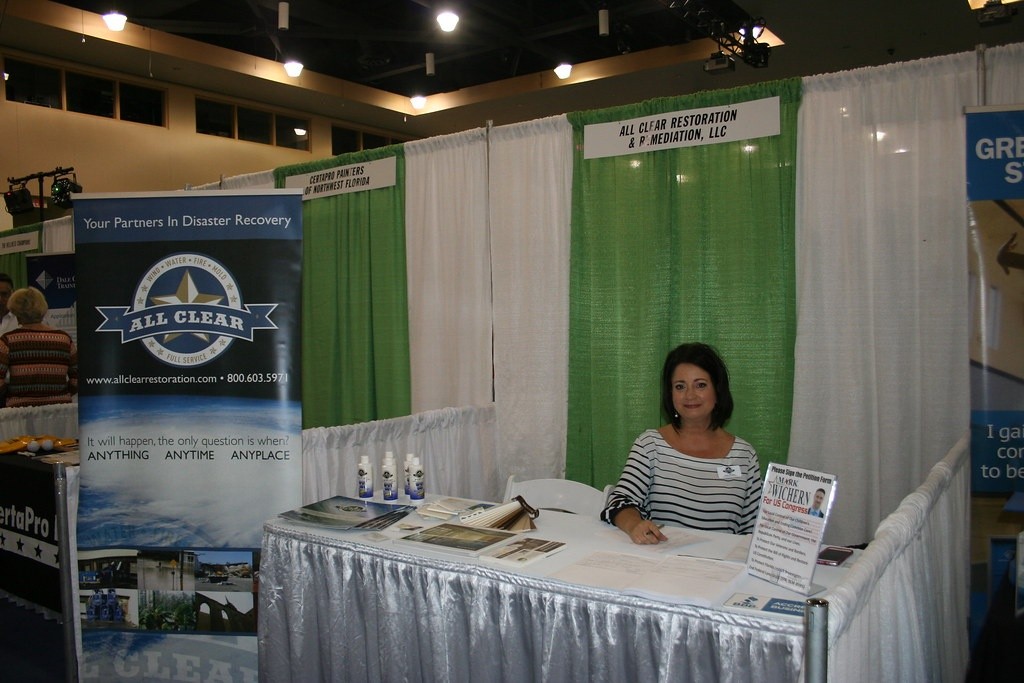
[284,62,305,77]
[3,182,35,216]
[743,43,771,68]
[704,51,737,76]
[51,171,82,209]
[435,12,460,32]
[553,63,572,80]
[103,3,127,33]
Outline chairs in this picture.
[502,475,614,521]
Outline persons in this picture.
[86,588,131,623]
[600,342,762,545]
[0,272,78,408]
[808,488,825,518]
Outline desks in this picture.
[258,492,870,682]
[0,444,80,627]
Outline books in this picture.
[479,536,568,569]
[415,498,477,521]
[277,496,418,532]
[393,521,526,557]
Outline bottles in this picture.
[358,455,373,498]
[409,457,425,503]
[383,451,396,465]
[382,460,398,501]
[404,454,415,496]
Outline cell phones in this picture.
[816,546,853,565]
